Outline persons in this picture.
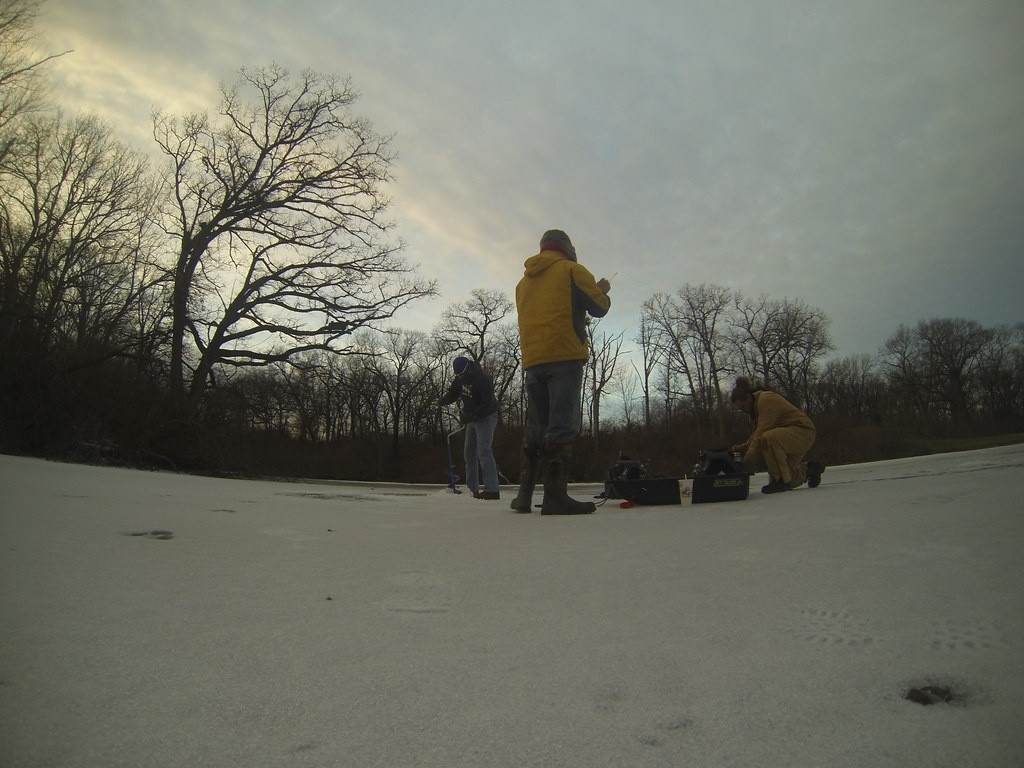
[728,377,825,494]
[434,356,501,501]
[510,229,613,515]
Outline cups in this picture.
[677,477,695,505]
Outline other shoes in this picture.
[806,461,825,488]
[470,490,500,499]
[761,477,793,494]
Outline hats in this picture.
[453,357,468,376]
[539,230,573,248]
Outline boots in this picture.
[509,440,543,513]
[541,444,596,515]
